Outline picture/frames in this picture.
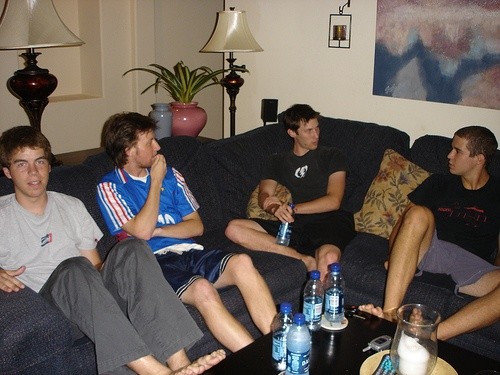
[328,13,352,48]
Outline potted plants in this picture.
[121,60,250,138]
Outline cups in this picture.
[390,304,442,375]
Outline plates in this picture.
[320,315,348,331]
[359,350,458,375]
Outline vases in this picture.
[149,103,172,139]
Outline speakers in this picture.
[261,99,278,122]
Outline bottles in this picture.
[152,104,172,140]
[302,271,324,330]
[285,313,312,375]
[275,204,295,246]
[271,303,294,361]
[325,263,346,321]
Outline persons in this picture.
[225,103,352,296]
[358,126,500,341]
[0,125,227,375]
[96,112,283,352]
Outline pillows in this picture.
[246,180,295,221]
[353,149,433,240]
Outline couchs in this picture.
[340,135,500,361]
[0,113,411,375]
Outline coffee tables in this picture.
[199,304,500,375]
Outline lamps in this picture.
[0,0,85,167]
[199,7,264,137]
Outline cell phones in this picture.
[361,334,393,352]
